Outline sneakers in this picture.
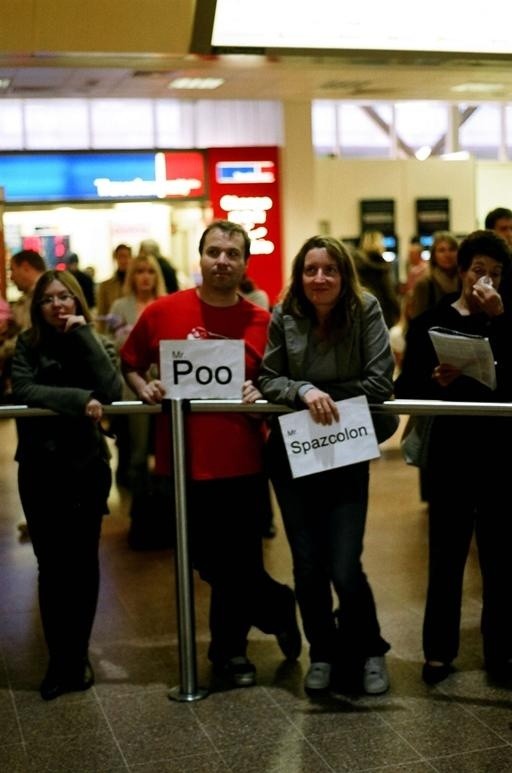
[213,588,390,693]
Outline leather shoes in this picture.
[41,662,94,701]
[422,661,449,684]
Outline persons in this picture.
[9,271,123,700]
[393,230,512,686]
[256,236,394,694]
[119,222,300,687]
